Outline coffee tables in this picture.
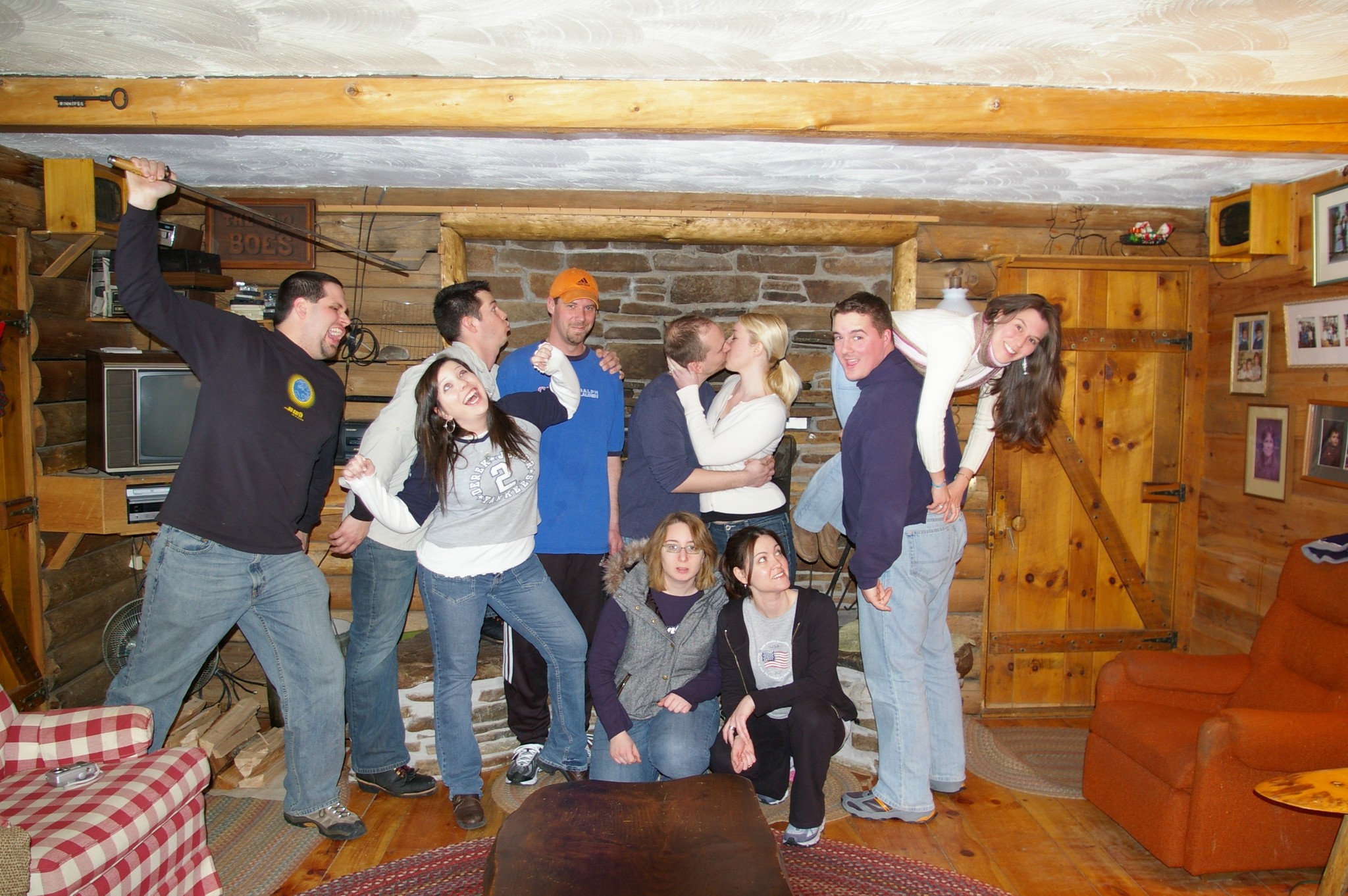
[483,773,793,896]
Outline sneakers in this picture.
[789,505,818,562]
[756,789,788,804]
[782,816,826,847]
[930,778,965,793]
[284,800,367,840]
[817,521,840,567]
[584,734,594,763]
[505,743,545,785]
[841,789,936,824]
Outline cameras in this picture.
[45,761,95,787]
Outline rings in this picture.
[956,506,960,509]
[938,505,943,508]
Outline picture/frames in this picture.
[1312,182,1348,287]
[1243,404,1290,502]
[1283,296,1348,368]
[1229,312,1270,396]
[1301,399,1348,489]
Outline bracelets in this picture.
[931,480,946,488]
[956,473,969,482]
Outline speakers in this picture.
[1209,185,1292,260]
[43,158,127,234]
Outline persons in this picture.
[101,155,367,841]
[827,292,968,823]
[342,340,591,832]
[331,263,805,799]
[715,527,861,849]
[583,510,731,785]
[791,293,1064,568]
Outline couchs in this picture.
[0,686,223,896]
[1078,539,1348,877]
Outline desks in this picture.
[1255,768,1348,896]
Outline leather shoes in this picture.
[480,616,504,644]
[536,758,589,781]
[356,764,437,797]
[452,793,486,830]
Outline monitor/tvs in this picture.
[86,349,201,477]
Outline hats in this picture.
[550,267,599,311]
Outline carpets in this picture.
[204,773,350,896]
[295,830,1013,895]
[962,717,1089,799]
